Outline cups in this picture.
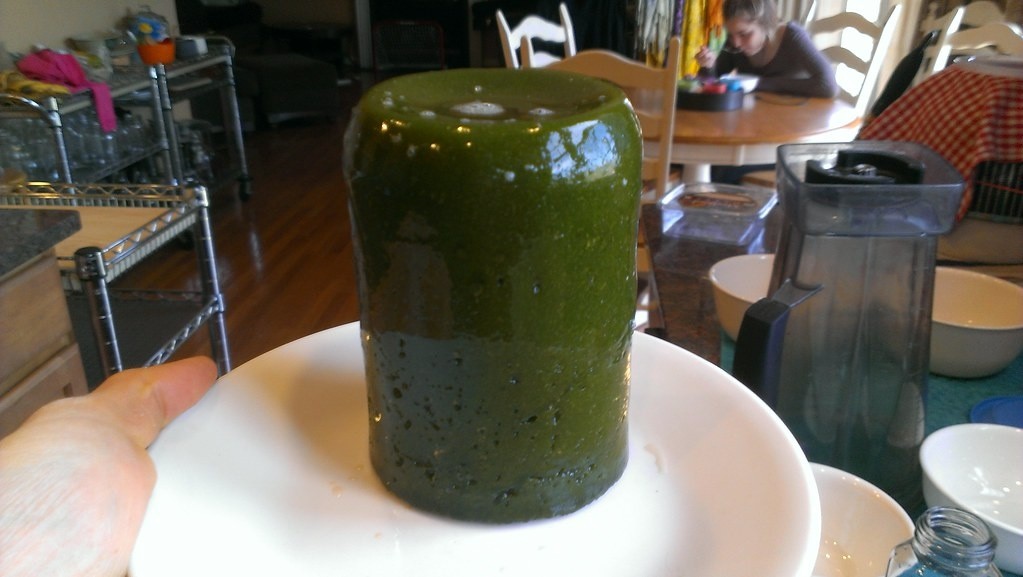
[0,107,152,181]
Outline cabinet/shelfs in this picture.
[1,11,250,223]
[0,165,232,387]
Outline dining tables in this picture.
[638,194,1023,577]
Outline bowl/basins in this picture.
[919,422,1023,577]
[871,265,1023,378]
[674,80,745,111]
[709,251,835,347]
[720,74,760,94]
[798,461,916,575]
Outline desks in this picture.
[587,72,863,245]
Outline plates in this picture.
[123,313,823,577]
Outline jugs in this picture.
[730,135,968,511]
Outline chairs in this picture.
[516,32,684,341]
[494,4,577,72]
[802,1,900,118]
[909,1,1023,97]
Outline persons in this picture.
[0,351,220,577]
[692,0,838,97]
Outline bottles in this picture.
[886,508,1005,577]
